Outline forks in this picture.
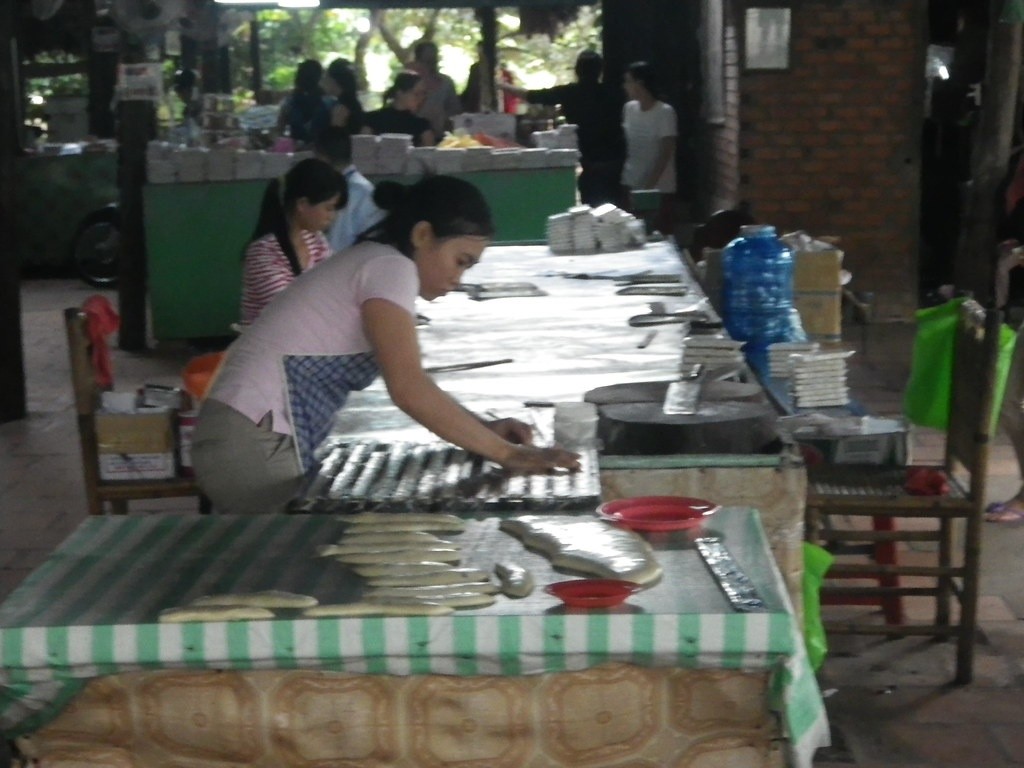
[662,362,704,415]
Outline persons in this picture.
[362,70,434,145]
[619,62,677,231]
[194,176,583,514]
[239,159,349,323]
[415,41,459,137]
[494,51,625,204]
[313,128,381,252]
[172,54,204,117]
[460,42,520,113]
[316,59,365,163]
[983,148,1024,523]
[276,60,336,144]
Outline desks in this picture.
[284,238,808,653]
[0,504,831,768]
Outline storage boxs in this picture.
[704,232,843,342]
[95,404,177,481]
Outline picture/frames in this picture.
[740,0,795,73]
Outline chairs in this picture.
[806,300,997,683]
[65,304,213,513]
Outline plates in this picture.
[542,579,644,609]
[596,496,723,533]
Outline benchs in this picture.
[759,368,906,640]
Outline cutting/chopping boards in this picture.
[583,378,777,455]
